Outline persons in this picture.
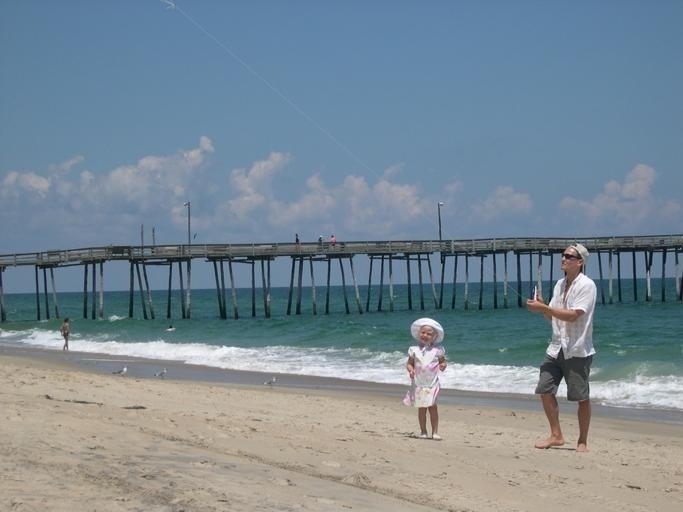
[328,234,336,253]
[523,241,599,454]
[317,235,322,253]
[294,233,300,253]
[402,317,447,441]
[58,316,70,352]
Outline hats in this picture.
[410,317,445,344]
[570,243,590,273]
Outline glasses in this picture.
[562,254,579,259]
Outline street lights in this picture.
[436,200,444,241]
[183,200,191,254]
